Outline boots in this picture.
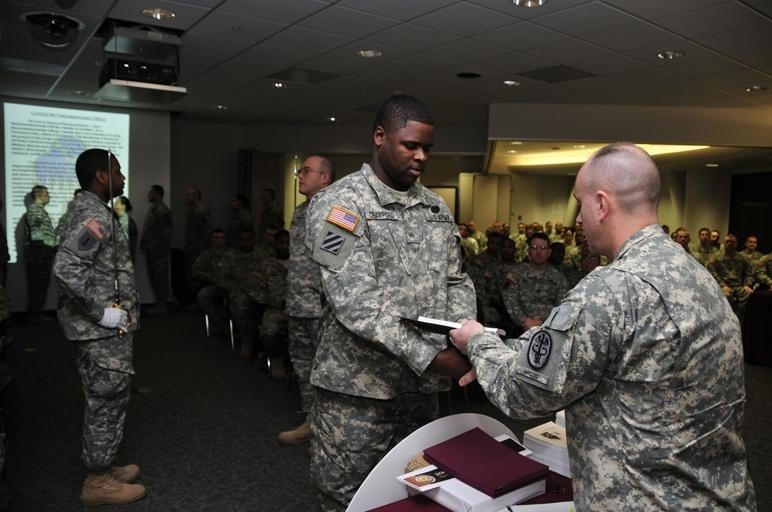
[278,419,314,446]
[79,473,146,507]
[29,315,55,324]
[105,464,140,481]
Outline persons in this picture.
[467,220,487,252]
[707,235,754,320]
[708,231,724,252]
[182,186,289,378]
[111,193,137,264]
[26,184,61,325]
[305,95,477,509]
[450,142,756,506]
[671,232,677,244]
[738,236,762,269]
[455,222,479,254]
[756,250,772,294]
[53,148,147,502]
[504,233,567,335]
[144,184,178,312]
[696,229,714,262]
[675,227,705,265]
[497,237,518,270]
[470,230,511,331]
[278,156,335,447]
[563,214,601,286]
[489,219,566,236]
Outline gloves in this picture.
[97,307,130,333]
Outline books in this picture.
[424,427,551,497]
[398,315,505,337]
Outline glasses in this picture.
[530,245,550,252]
[296,167,324,176]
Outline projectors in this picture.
[100,58,176,88]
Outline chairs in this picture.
[230,299,264,351]
[347,414,520,511]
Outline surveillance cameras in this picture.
[26,13,78,52]
